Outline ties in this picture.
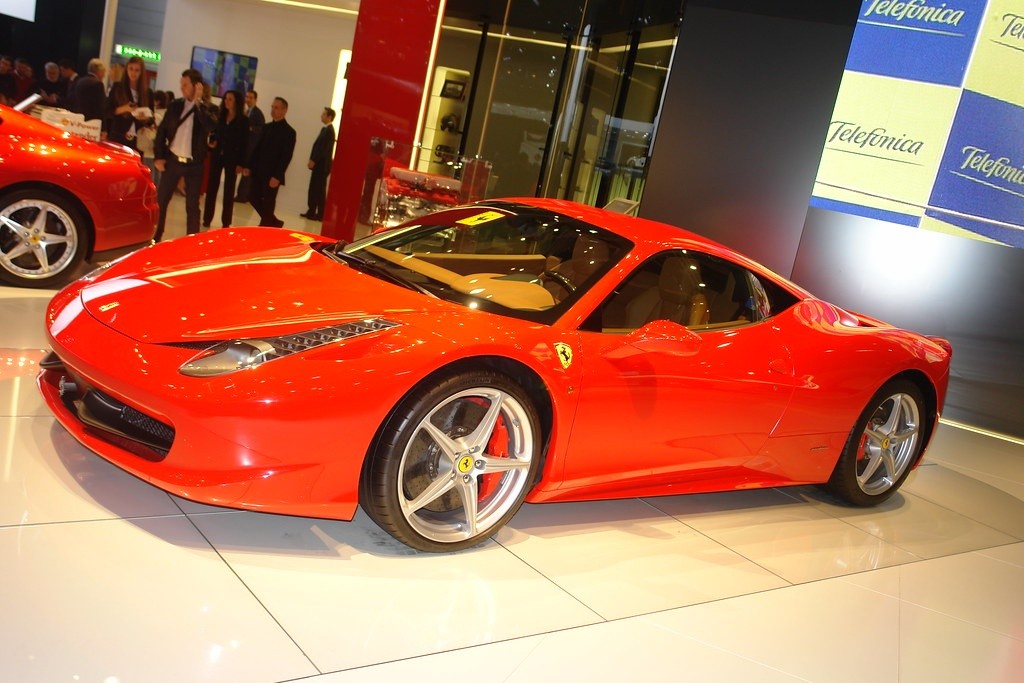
[245,109,249,116]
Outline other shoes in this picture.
[221,223,230,228]
[259,216,284,229]
[300,208,322,222]
[203,222,211,227]
[233,194,248,203]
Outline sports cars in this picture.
[34,196,953,554]
[0,104,161,288]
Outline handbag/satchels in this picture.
[136,119,159,153]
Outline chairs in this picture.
[540,232,712,340]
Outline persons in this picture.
[299,107,336,223]
[203,89,248,232]
[234,89,266,204]
[243,97,297,229]
[147,67,211,239]
[0,52,215,195]
